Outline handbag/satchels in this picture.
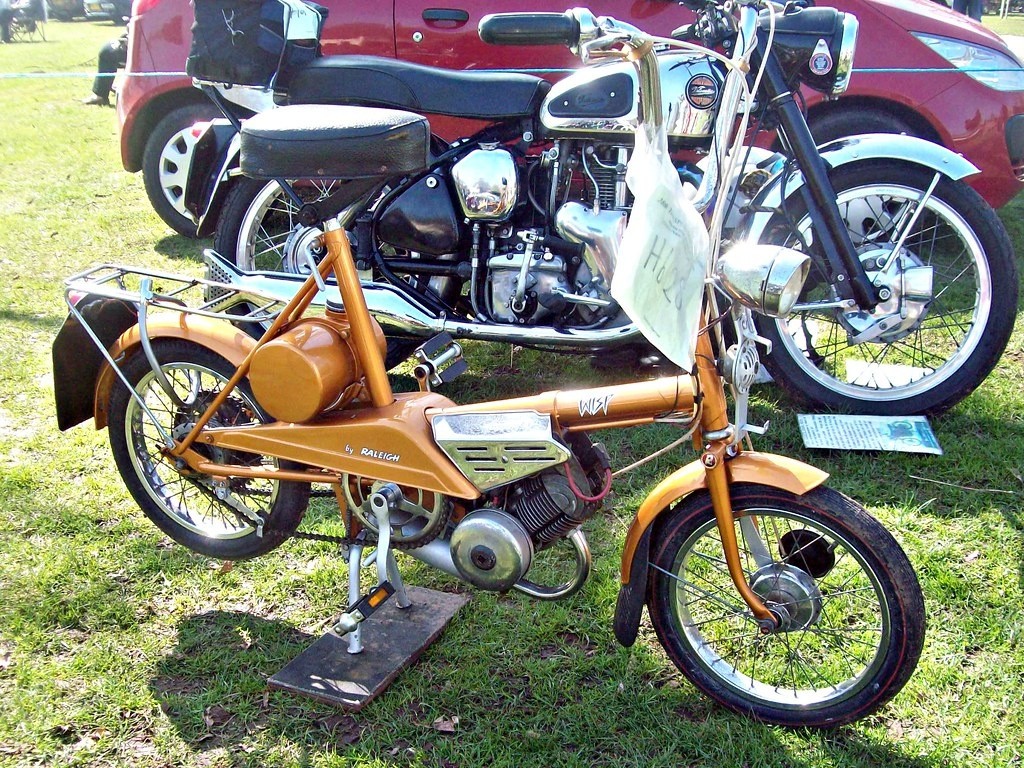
[609,122,710,377]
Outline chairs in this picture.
[9,0,48,43]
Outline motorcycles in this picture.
[179,0,1020,423]
[42,0,928,728]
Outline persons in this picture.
[0,6,19,42]
[81,34,127,102]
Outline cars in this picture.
[121,1,1022,281]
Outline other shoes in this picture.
[80,95,110,104]
